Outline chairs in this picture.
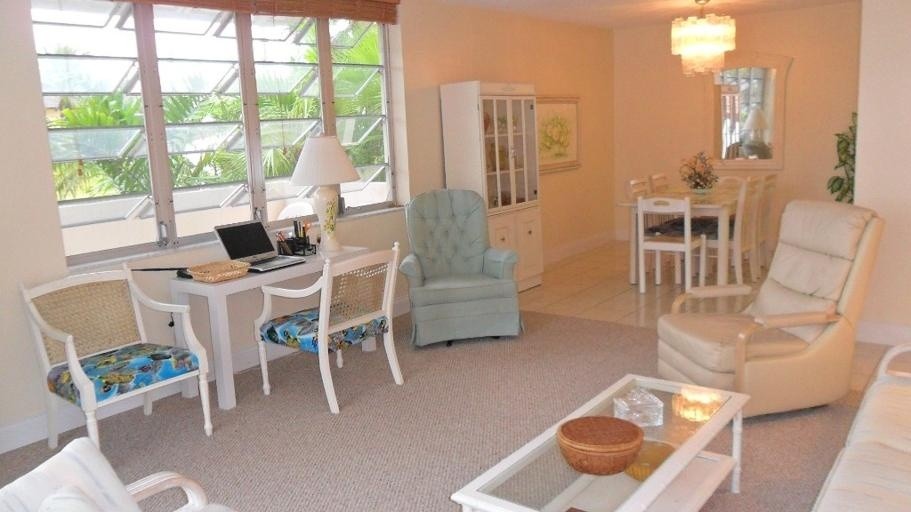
[618,169,780,288]
[0,434,241,512]
[16,261,215,460]
[654,198,886,430]
[398,187,527,350]
[253,239,406,415]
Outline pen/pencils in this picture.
[294,221,306,237]
[276,231,293,254]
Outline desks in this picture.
[171,246,379,411]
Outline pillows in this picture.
[2,435,146,512]
[38,484,99,512]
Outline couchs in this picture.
[809,343,911,511]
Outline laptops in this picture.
[214,218,306,273]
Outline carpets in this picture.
[2,305,865,511]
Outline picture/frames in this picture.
[536,94,586,174]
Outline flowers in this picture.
[678,149,720,190]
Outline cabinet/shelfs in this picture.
[438,80,546,294]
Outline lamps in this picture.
[289,135,362,254]
[741,108,770,142]
[668,0,739,79]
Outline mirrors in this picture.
[712,51,795,179]
